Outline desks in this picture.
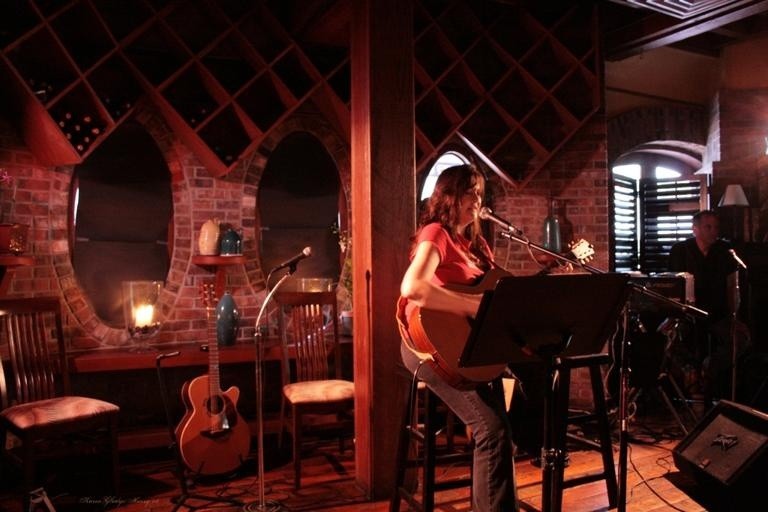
[74,335,353,450]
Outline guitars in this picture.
[408,237,595,391]
[175,277,250,476]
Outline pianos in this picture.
[547,271,695,310]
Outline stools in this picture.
[511,351,619,512]
[389,375,515,512]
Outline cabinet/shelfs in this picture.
[0,0,351,172]
[413,0,602,188]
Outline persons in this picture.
[668,210,752,391]
[397,162,574,512]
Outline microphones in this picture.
[477,205,525,234]
[271,245,314,274]
[726,247,747,270]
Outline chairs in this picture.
[276,291,355,489]
[0,296,122,512]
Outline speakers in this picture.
[671,398,768,488]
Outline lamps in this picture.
[718,184,750,240]
